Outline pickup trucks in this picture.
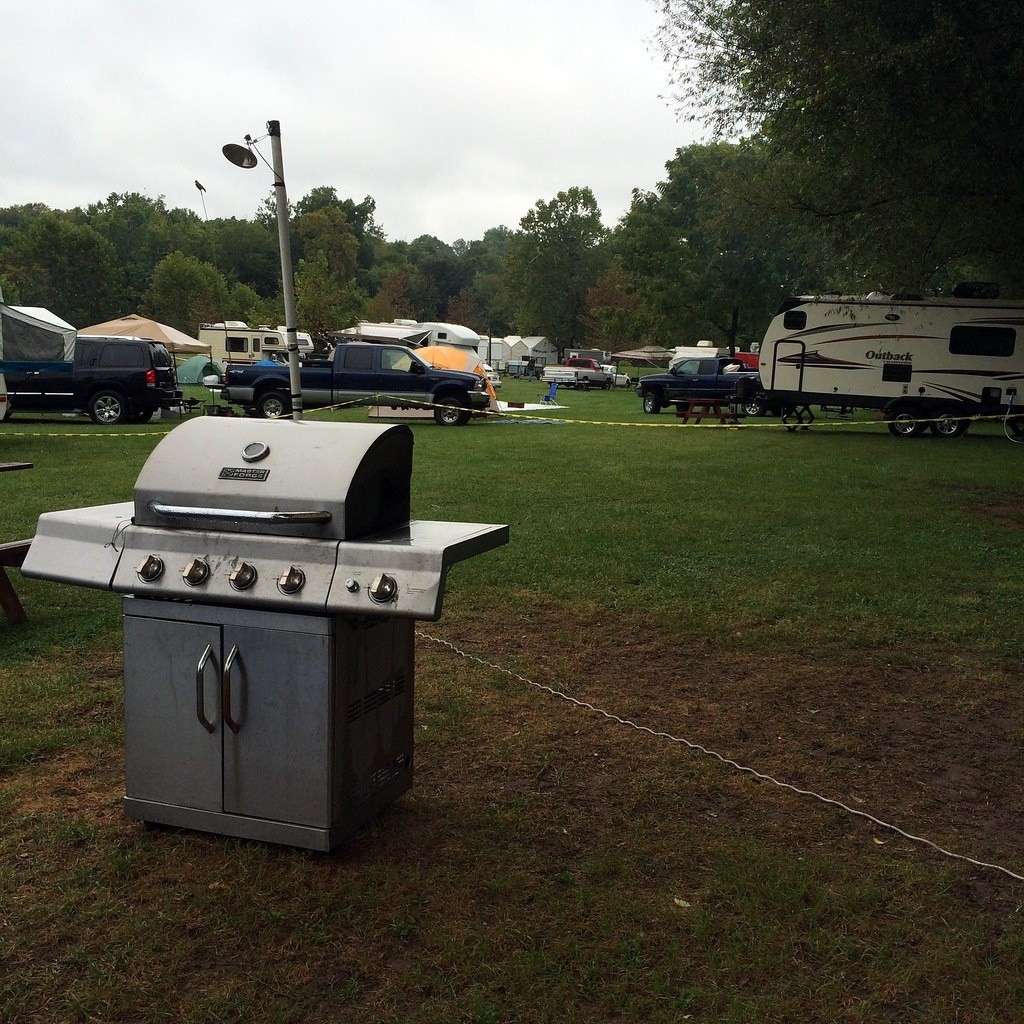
[220,342,493,428]
[541,358,614,391]
[637,356,771,417]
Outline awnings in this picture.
[325,323,433,349]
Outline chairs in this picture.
[383,355,392,369]
[537,383,558,405]
[629,377,640,392]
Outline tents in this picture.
[76,313,214,419]
[175,355,225,385]
[503,334,529,365]
[610,345,676,390]
[365,345,503,418]
[498,339,510,365]
[0,301,77,360]
[524,333,558,367]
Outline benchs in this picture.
[0,538,36,567]
[676,412,746,419]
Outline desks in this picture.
[0,461,34,623]
[182,399,207,413]
[680,398,746,424]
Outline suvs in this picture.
[1,337,186,425]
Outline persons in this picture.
[527,358,535,381]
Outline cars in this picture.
[600,363,631,387]
[482,362,504,389]
